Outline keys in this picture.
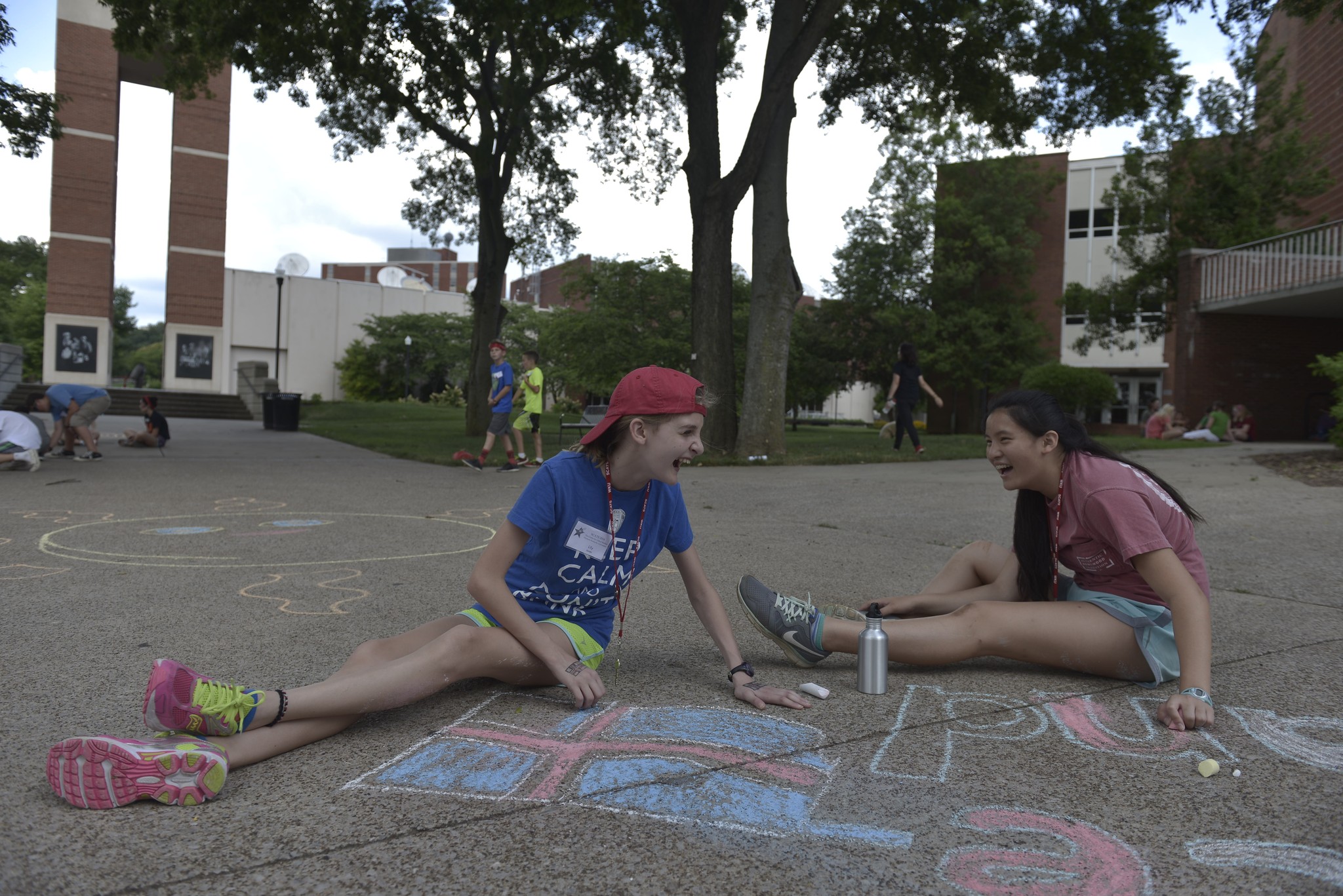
[615,657,620,685]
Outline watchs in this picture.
[727,661,754,683]
[1180,687,1213,707]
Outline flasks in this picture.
[858,602,889,695]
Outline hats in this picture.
[580,365,708,446]
[143,397,158,410]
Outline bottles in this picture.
[882,399,896,413]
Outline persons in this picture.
[732,390,1218,731]
[27,382,111,461]
[1139,395,1259,444]
[43,365,816,807]
[512,351,544,467]
[119,395,171,448]
[886,343,944,454]
[461,338,521,473]
[0,405,49,472]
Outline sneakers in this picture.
[46,731,229,808]
[496,462,519,472]
[142,659,267,736]
[736,574,834,668]
[461,459,484,472]
[815,603,900,622]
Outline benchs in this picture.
[558,404,608,445]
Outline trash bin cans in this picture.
[273,392,302,431]
[261,392,278,429]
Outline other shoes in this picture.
[27,448,41,472]
[73,450,103,462]
[523,458,544,467]
[118,434,134,447]
[505,454,528,466]
[915,444,924,453]
[45,448,75,458]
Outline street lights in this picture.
[273,263,286,381]
